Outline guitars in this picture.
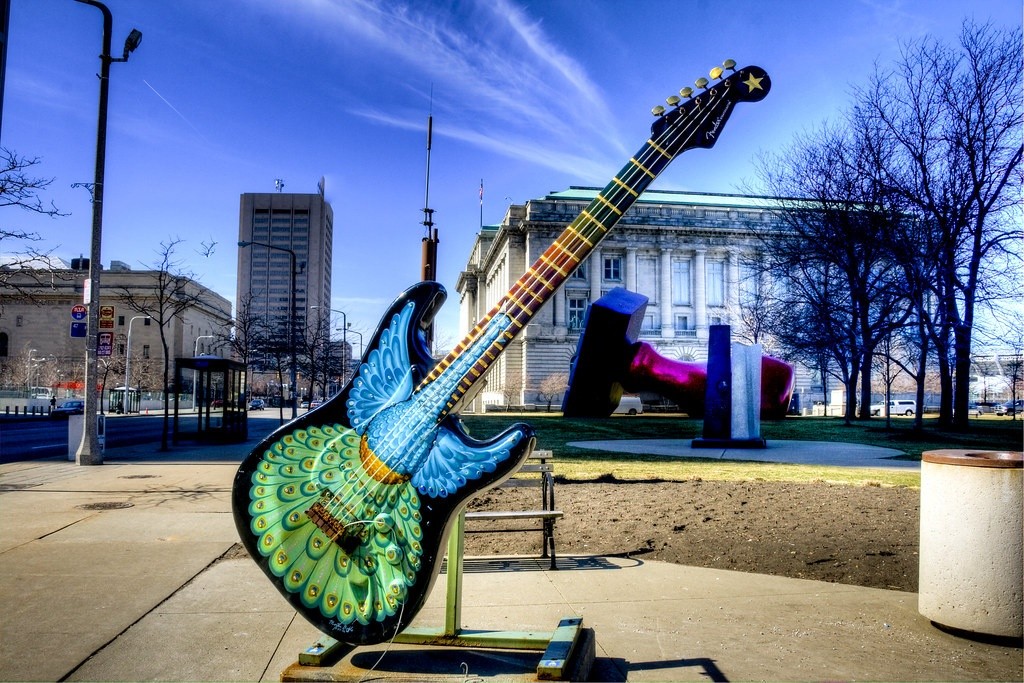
[231,57,772,647]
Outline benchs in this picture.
[465,449,564,570]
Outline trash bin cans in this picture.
[920,450,1024,639]
[69,415,107,462]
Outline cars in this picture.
[300,400,321,409]
[994,399,1024,417]
[50,400,85,415]
[612,395,643,416]
[248,399,265,411]
[870,398,917,417]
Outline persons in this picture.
[50,395,57,408]
[117,398,123,410]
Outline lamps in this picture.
[15,315,23,327]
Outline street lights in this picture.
[28,349,37,392]
[236,239,308,419]
[123,316,154,415]
[32,357,46,387]
[310,305,352,389]
[336,328,363,359]
[70,0,143,468]
[192,335,216,414]
